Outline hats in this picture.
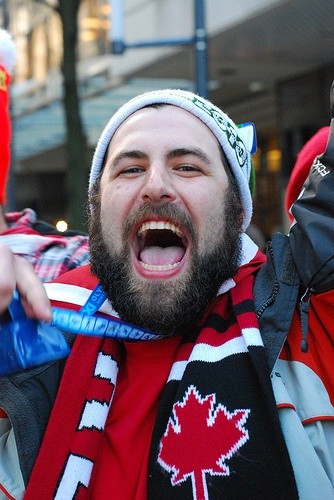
[286,127,331,222]
[88,89,252,232]
[0,29,17,205]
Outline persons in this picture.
[0,88,334,500]
